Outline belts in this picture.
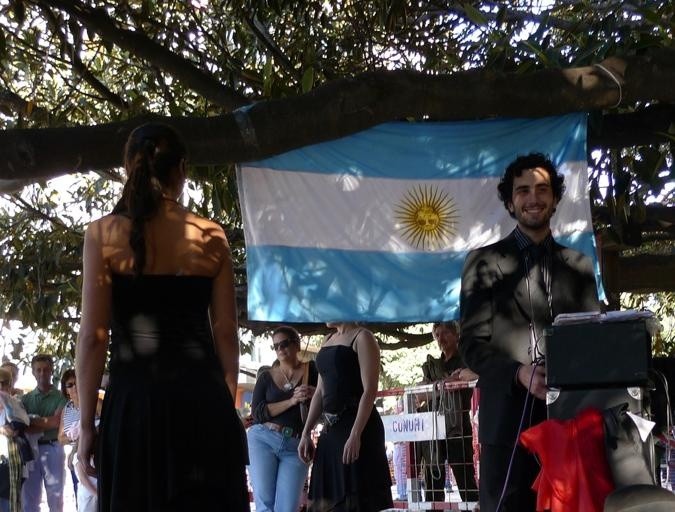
[264,422,302,441]
[38,438,58,445]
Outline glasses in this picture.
[274,337,297,350]
[65,380,75,387]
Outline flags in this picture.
[234,114,611,323]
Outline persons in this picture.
[296,323,395,512]
[421,320,480,502]
[73,123,247,512]
[57,369,81,512]
[247,326,318,512]
[72,452,98,512]
[0,362,29,512]
[21,354,67,512]
[460,152,600,511]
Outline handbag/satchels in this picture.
[300,361,324,425]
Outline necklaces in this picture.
[278,362,299,391]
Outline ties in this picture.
[527,246,551,355]
